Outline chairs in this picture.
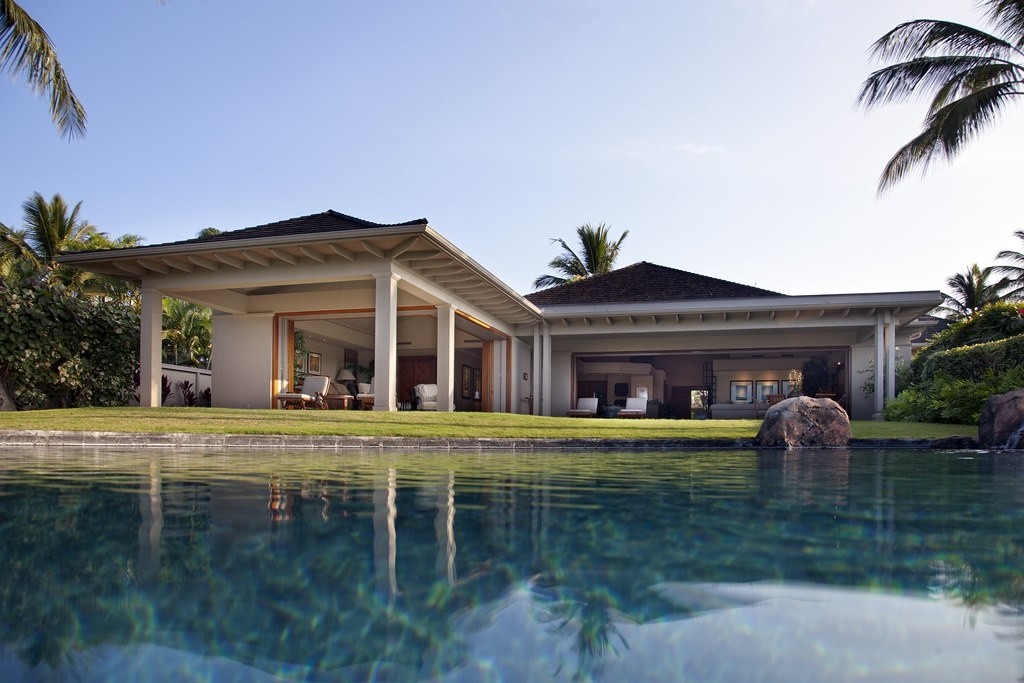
[337,369,356,394]
[277,374,331,409]
[567,397,598,417]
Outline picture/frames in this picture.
[294,348,305,372]
[635,385,648,400]
[781,379,804,398]
[462,364,472,398]
[730,379,753,403]
[307,350,322,375]
[755,379,779,401]
[473,367,482,400]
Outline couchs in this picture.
[411,383,455,411]
[294,375,374,409]
[617,397,646,418]
[710,402,771,420]
